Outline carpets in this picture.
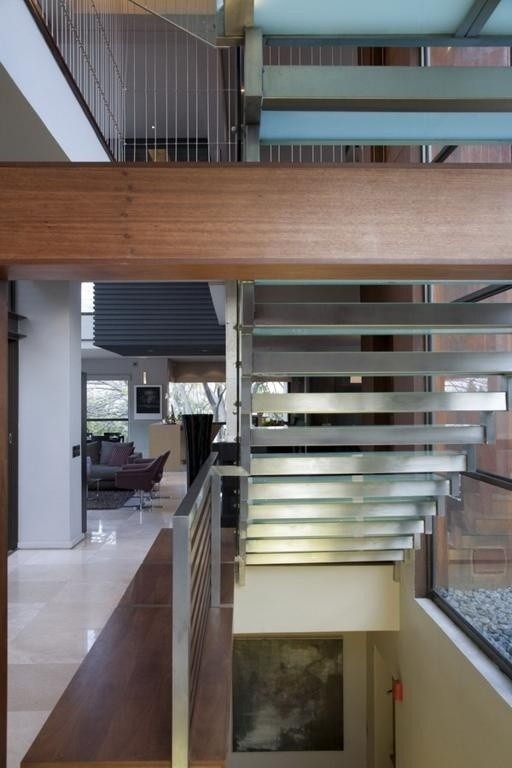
[438,586,512,666]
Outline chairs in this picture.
[86,441,171,512]
